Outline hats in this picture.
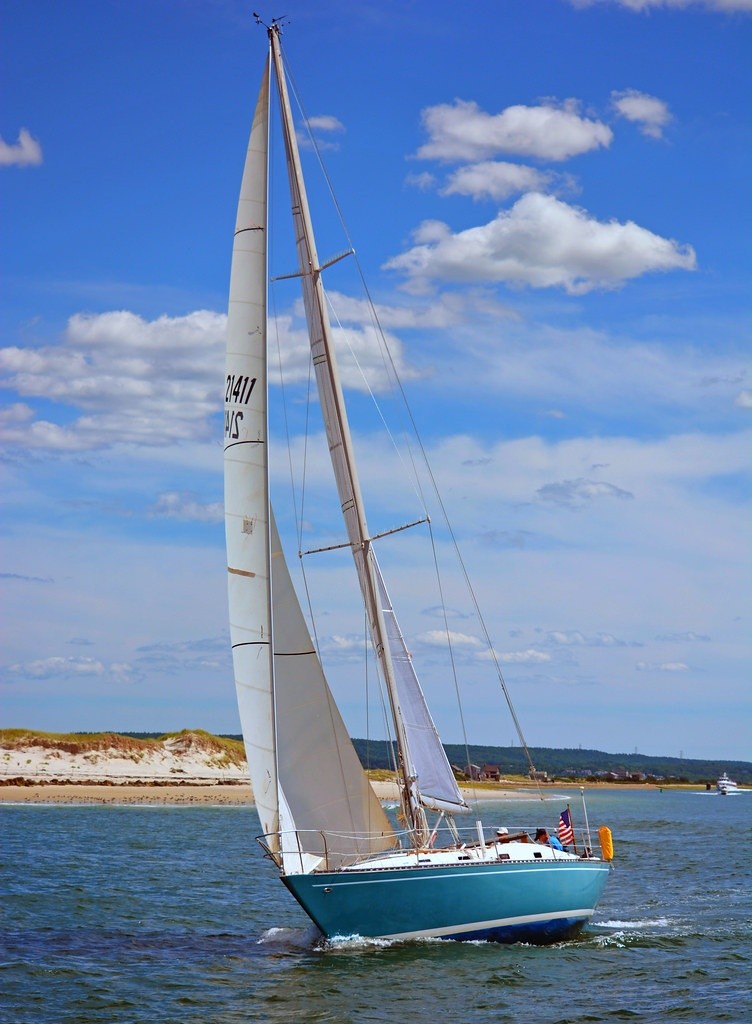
[497,827,508,834]
[534,829,547,841]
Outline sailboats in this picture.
[222,12,615,956]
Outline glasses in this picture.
[497,833,503,836]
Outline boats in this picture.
[715,772,738,792]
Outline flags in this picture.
[558,811,573,846]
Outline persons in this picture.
[534,829,563,851]
[490,827,517,846]
[582,848,592,858]
